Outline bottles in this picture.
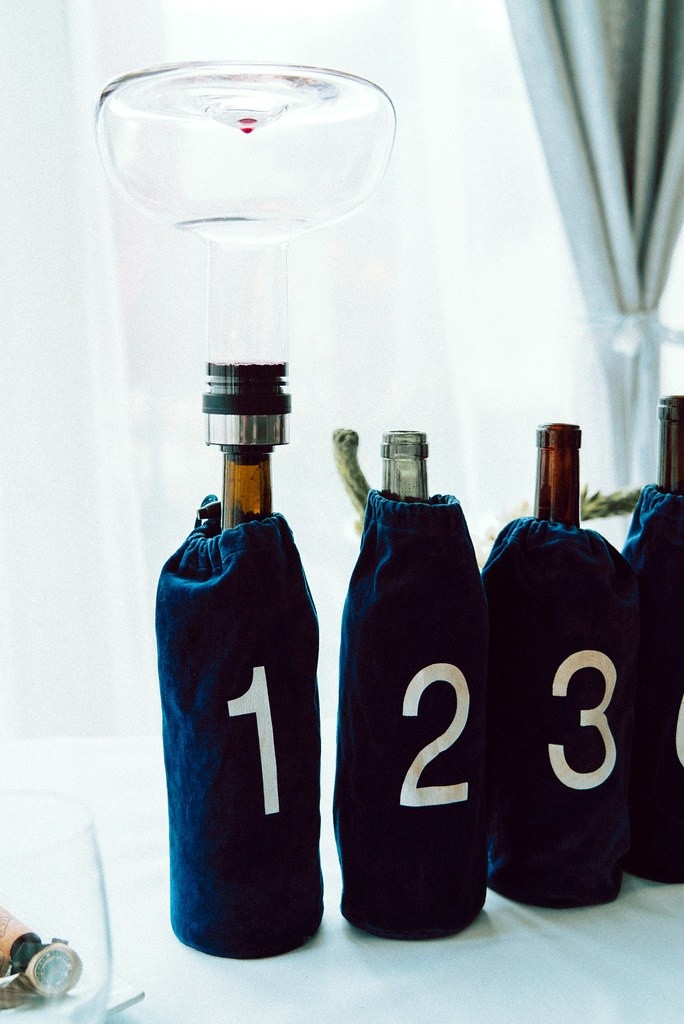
[203,360,289,536]
[654,392,683,496]
[530,424,583,528]
[374,428,430,505]
